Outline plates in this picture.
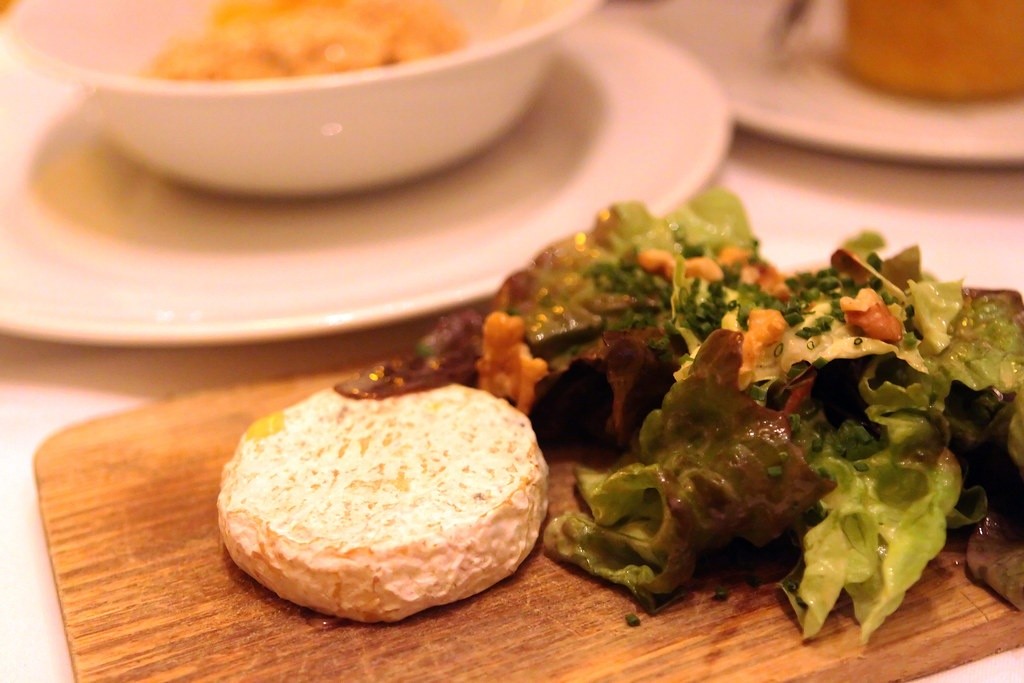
[0,1,734,349]
[640,1,1024,169]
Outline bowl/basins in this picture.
[11,1,599,193]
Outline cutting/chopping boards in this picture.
[33,364,1024,683]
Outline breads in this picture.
[217,380,549,623]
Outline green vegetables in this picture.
[334,183,1024,647]
